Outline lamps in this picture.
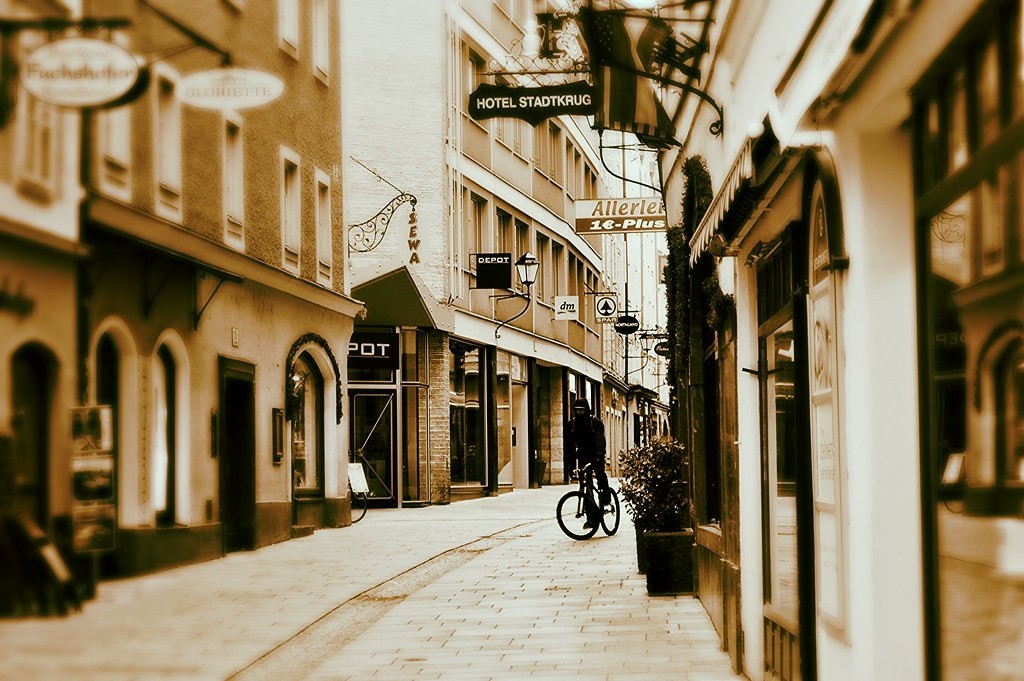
[514,252,541,287]
[639,332,654,350]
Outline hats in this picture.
[573,398,589,409]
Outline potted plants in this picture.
[617,435,677,574]
[625,440,694,596]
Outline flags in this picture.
[592,11,678,143]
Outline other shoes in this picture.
[583,522,593,529]
[604,505,611,512]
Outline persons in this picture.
[561,398,611,526]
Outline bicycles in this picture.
[556,461,621,540]
[348,478,367,523]
